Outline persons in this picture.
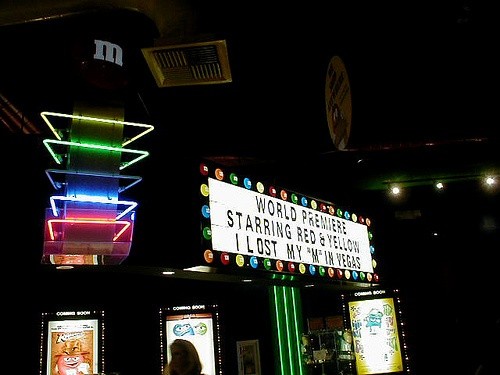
[162,338,207,375]
